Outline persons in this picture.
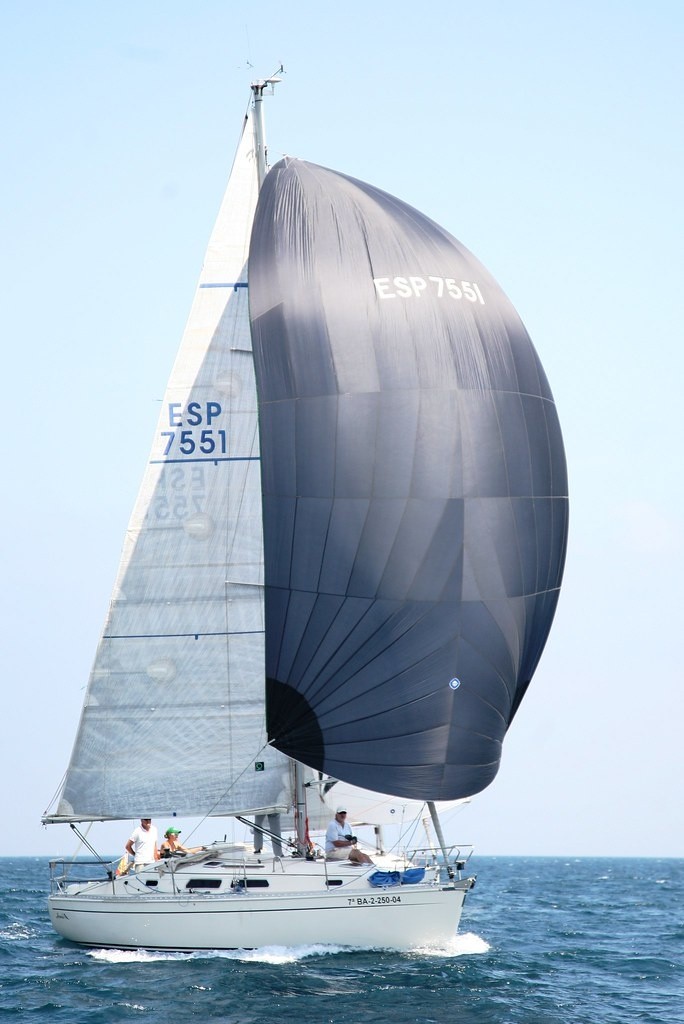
[253,813,285,857]
[325,807,373,864]
[125,819,158,874]
[160,827,203,860]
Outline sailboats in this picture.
[36,62,572,960]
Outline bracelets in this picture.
[350,841,353,845]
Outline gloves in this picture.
[345,835,358,844]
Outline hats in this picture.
[336,806,348,813]
[165,827,181,837]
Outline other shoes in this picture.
[254,850,261,853]
